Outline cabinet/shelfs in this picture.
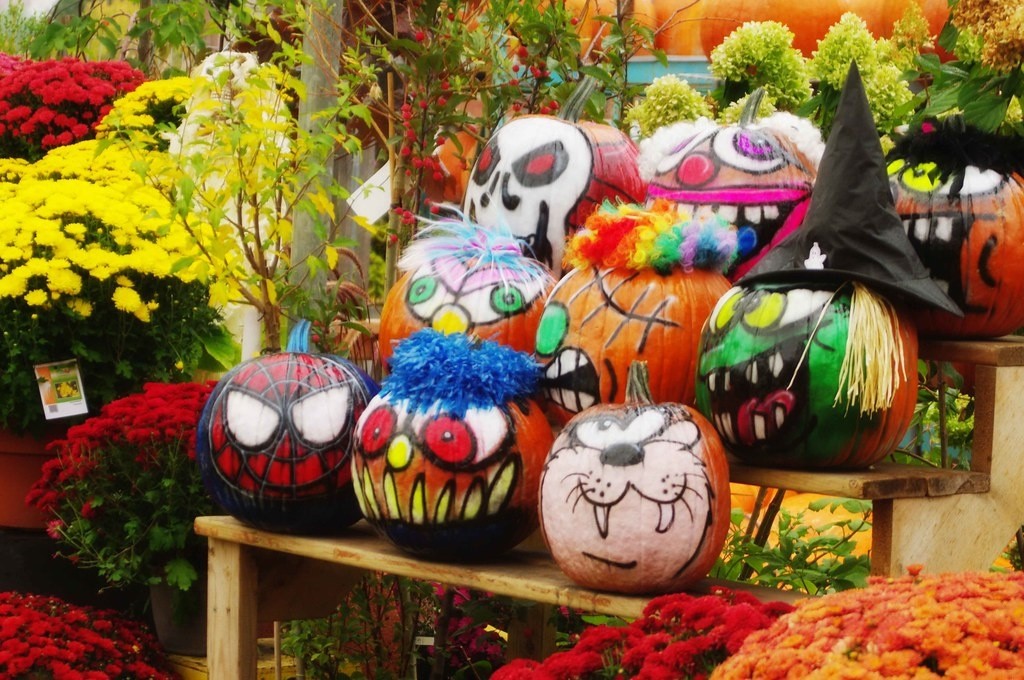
[187,326,1024,680]
